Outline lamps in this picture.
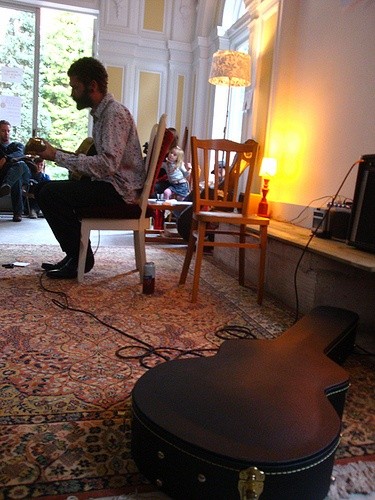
[207,48,251,182]
[257,158,278,218]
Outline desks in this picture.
[146,198,193,245]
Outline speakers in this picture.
[347,154,375,253]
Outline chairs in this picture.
[78,114,167,284]
[177,136,270,305]
[22,182,35,213]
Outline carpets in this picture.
[0,243,375,500]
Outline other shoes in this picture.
[29,210,44,219]
[41,246,95,279]
[13,215,22,222]
[0,184,11,197]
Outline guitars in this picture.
[24,137,98,182]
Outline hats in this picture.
[211,161,225,174]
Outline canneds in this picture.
[143,262,155,294]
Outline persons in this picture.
[0,120,50,222]
[151,127,192,226]
[28,57,143,279]
[200,161,232,188]
